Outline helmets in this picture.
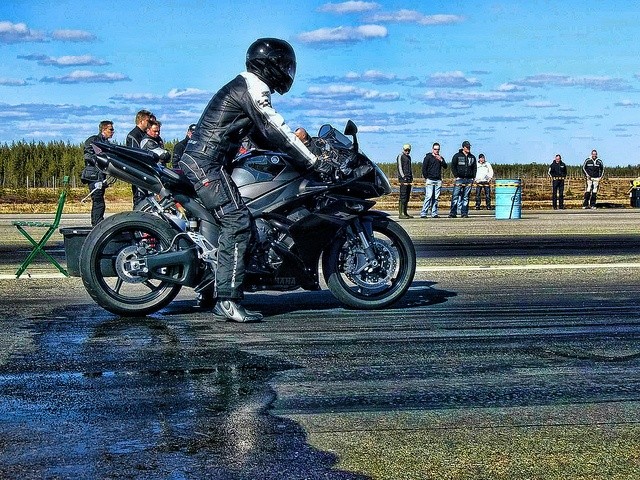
[245,38,296,96]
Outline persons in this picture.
[126,109,165,211]
[548,154,567,210]
[420,143,447,218]
[448,141,477,219]
[582,150,603,210]
[473,154,493,210]
[81,120,115,227]
[293,126,308,148]
[172,124,198,170]
[397,144,413,219]
[139,121,170,167]
[179,37,335,323]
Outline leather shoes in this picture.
[559,207,566,209]
[462,215,469,218]
[449,214,456,218]
[421,216,428,218]
[432,216,440,218]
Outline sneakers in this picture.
[476,207,480,210]
[196,292,214,309]
[214,300,263,324]
[590,206,596,209]
[583,206,589,209]
[487,207,491,209]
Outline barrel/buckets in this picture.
[495,179,520,219]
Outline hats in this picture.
[403,144,411,150]
[462,141,472,147]
[188,124,196,132]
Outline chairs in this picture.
[12,175,69,278]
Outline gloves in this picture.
[95,181,102,190]
[312,159,352,183]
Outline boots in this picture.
[405,201,413,219]
[399,201,409,219]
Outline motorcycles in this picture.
[78,119,417,316]
[135,195,177,218]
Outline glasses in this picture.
[405,150,411,152]
[434,149,440,150]
[108,128,113,132]
